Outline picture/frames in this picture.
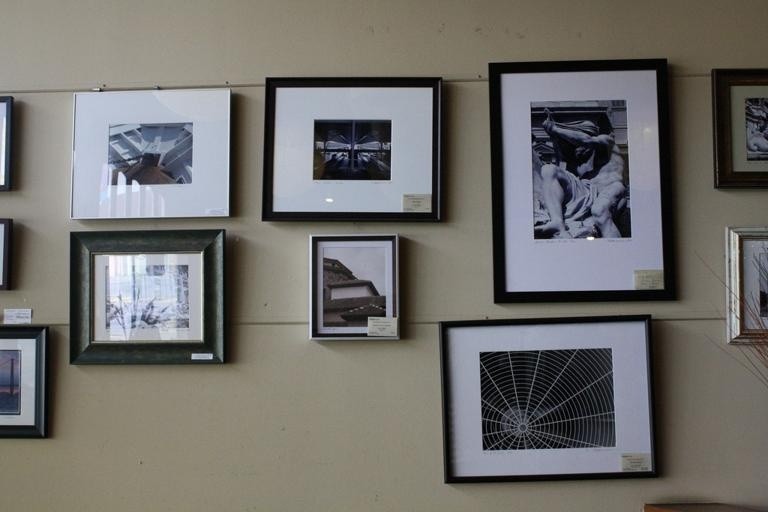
[69,88,230,219]
[68,229,228,365]
[439,314,660,483]
[261,75,449,224]
[487,56,679,304]
[0,95,15,192]
[0,218,13,291]
[308,232,399,341]
[711,67,768,189]
[725,225,768,345]
[0,324,53,439]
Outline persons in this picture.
[531,109,628,240]
